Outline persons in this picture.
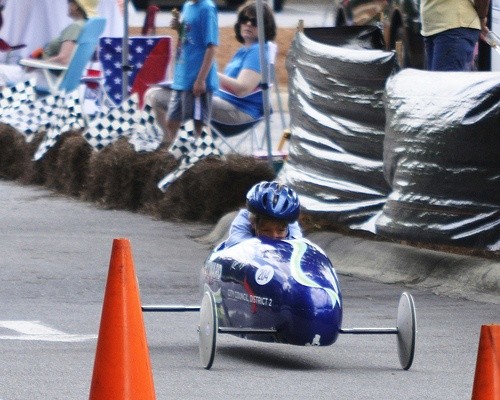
[155,0,219,152]
[0,0,99,93]
[420,0,489,72]
[226,181,303,250]
[143,1,271,152]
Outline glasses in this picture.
[239,16,257,26]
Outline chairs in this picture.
[81,35,171,115]
[199,40,287,156]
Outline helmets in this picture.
[246,181,300,224]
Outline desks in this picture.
[20,57,68,94]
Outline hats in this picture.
[75,0,98,19]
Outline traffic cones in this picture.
[470,324,500,400]
[87,237,158,400]
